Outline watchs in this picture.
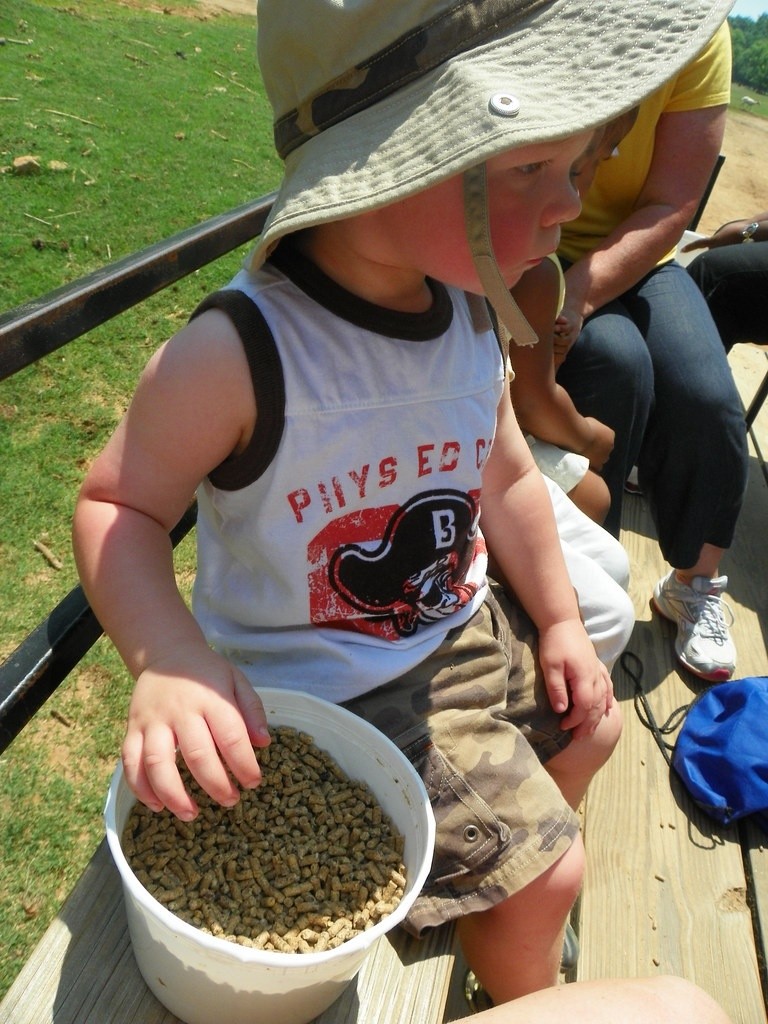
[741,221,759,243]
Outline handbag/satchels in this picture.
[671,673,768,828]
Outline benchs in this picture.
[0,189,457,1024]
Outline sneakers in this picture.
[653,569,738,682]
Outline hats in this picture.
[253,2,736,268]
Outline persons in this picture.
[509,95,646,529]
[682,211,768,359]
[555,21,752,681]
[68,1,736,1014]
[545,476,638,687]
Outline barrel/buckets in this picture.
[102,686,439,1023]
